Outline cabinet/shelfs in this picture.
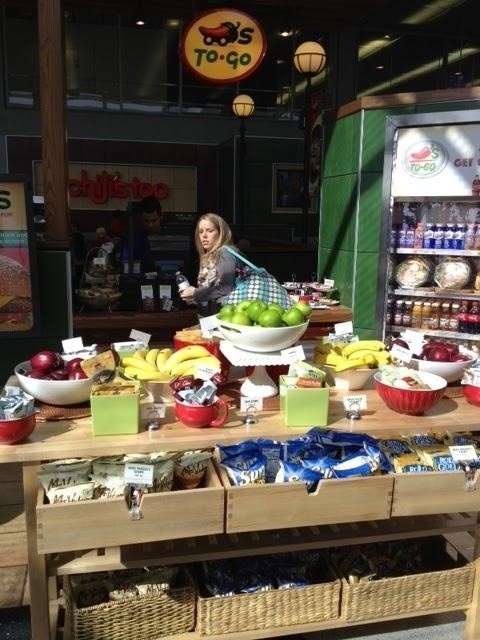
[374,109,480,388]
[0,375,480,640]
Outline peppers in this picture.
[199,22,239,42]
[410,147,431,160]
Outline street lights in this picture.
[230,94,257,225]
[286,39,330,244]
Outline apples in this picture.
[393,339,469,362]
[218,300,312,328]
[24,350,88,381]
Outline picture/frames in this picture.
[272,162,318,215]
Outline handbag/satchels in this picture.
[220,268,295,311]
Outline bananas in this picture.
[121,344,221,382]
[315,340,392,373]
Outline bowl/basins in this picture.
[322,364,381,389]
[402,347,477,383]
[16,355,100,406]
[461,375,480,408]
[375,370,447,417]
[216,317,311,356]
[0,411,41,446]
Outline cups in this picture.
[177,397,227,429]
[120,259,145,279]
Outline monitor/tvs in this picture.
[146,234,194,276]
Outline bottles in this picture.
[387,296,480,335]
[176,272,197,306]
[397,221,480,251]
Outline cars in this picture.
[144,221,198,260]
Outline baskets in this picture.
[328,537,475,622]
[64,566,195,639]
[184,554,342,636]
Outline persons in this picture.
[181,213,238,323]
[125,196,177,263]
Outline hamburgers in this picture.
[0,255,34,333]
